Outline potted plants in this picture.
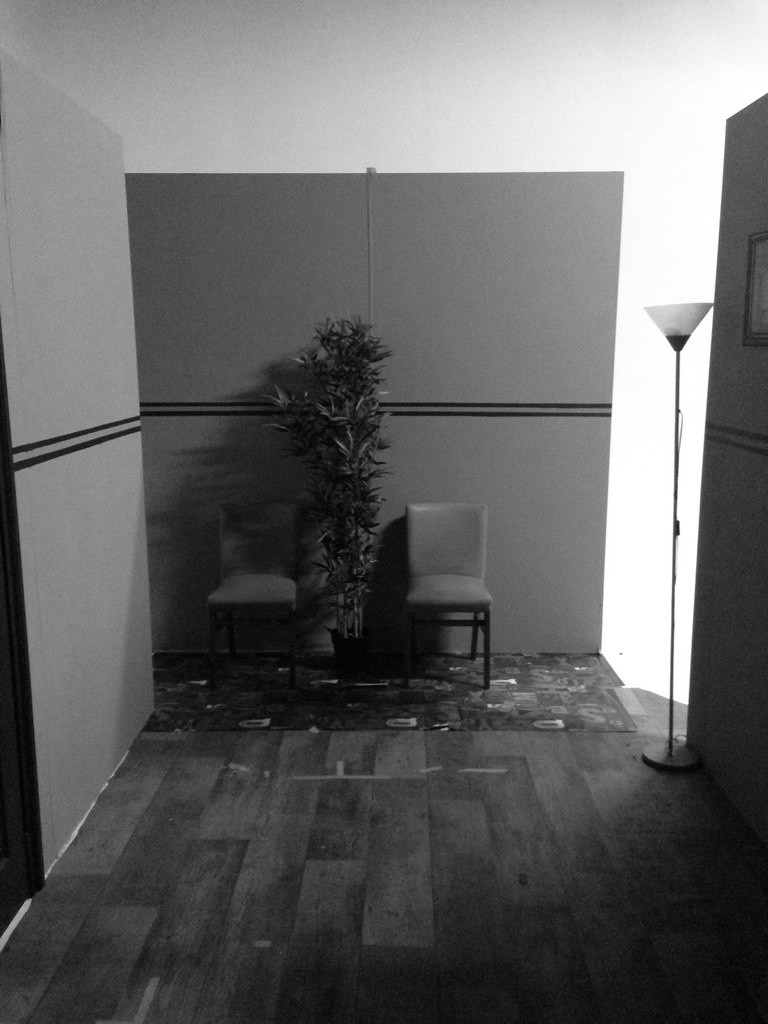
[258,313,395,686]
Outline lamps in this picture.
[642,303,716,774]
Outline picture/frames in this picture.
[742,231,768,346]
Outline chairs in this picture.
[205,499,303,691]
[400,502,493,690]
